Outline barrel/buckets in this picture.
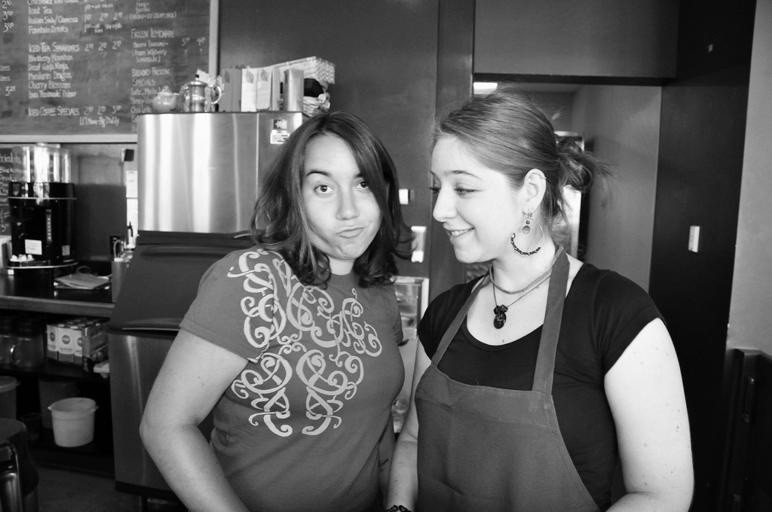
[47,397,99,448]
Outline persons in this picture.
[138,106,419,512]
[380,80,699,511]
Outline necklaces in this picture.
[490,265,551,329]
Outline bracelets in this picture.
[383,503,414,512]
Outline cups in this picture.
[184,74,222,113]
[153,93,183,113]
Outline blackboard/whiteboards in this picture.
[0,0,219,143]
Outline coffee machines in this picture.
[6,145,78,277]
